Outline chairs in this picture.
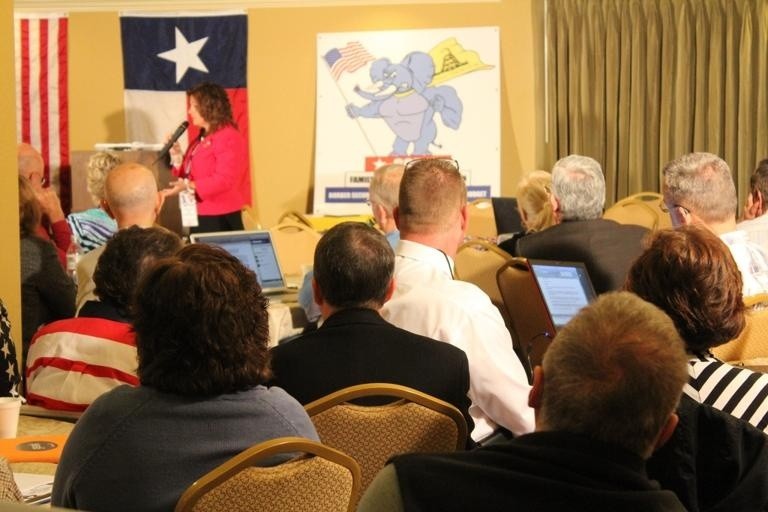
[706,293,768,377]
[494,253,554,382]
[605,200,659,235]
[304,382,468,500]
[600,191,674,230]
[463,196,523,246]
[455,240,513,345]
[173,437,360,512]
[268,222,322,289]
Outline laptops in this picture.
[526,258,598,330]
[190,230,300,302]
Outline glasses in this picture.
[35,176,48,187]
[529,332,556,380]
[404,157,460,170]
[659,199,690,215]
[366,198,375,207]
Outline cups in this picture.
[2,397,20,442]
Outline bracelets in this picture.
[184,176,196,196]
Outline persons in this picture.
[24,228,186,412]
[352,290,691,512]
[52,244,324,512]
[295,160,404,323]
[659,153,767,294]
[628,224,767,432]
[163,81,252,234]
[737,156,767,256]
[20,176,77,342]
[258,221,474,441]
[59,164,179,318]
[514,154,648,295]
[495,168,561,258]
[378,161,534,436]
[65,151,123,252]
[19,138,79,271]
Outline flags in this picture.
[18,12,72,213]
[407,38,494,84]
[325,41,373,82]
[116,11,251,164]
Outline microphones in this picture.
[157,119,190,162]
